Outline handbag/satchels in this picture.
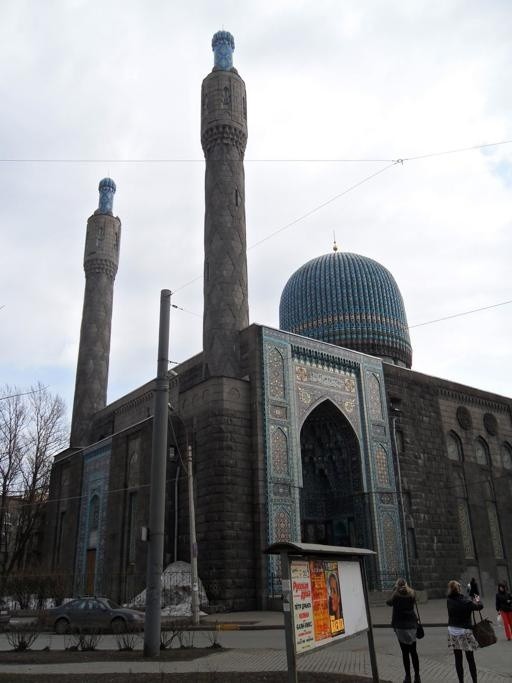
[415,624,425,639]
[470,617,498,648]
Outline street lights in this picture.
[167,401,200,627]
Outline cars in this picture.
[44,597,145,633]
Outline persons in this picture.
[446,577,512,683]
[385,578,425,683]
[328,574,343,621]
